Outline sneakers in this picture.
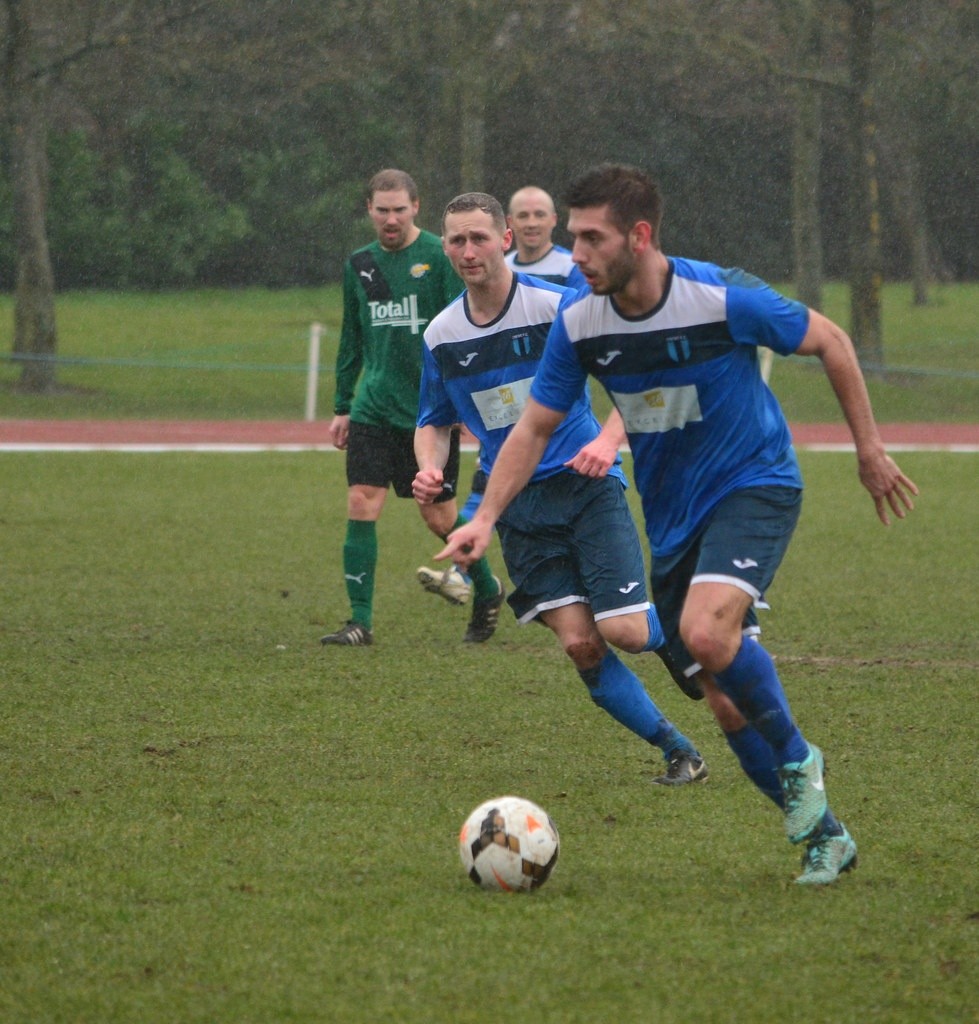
[320,619,373,645]
[771,740,828,843]
[651,750,709,787]
[792,822,858,883]
[653,640,706,700]
[416,564,469,603]
[461,575,506,642]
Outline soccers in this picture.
[458,796,561,894]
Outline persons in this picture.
[414,193,713,787]
[319,168,593,646]
[435,162,917,885]
